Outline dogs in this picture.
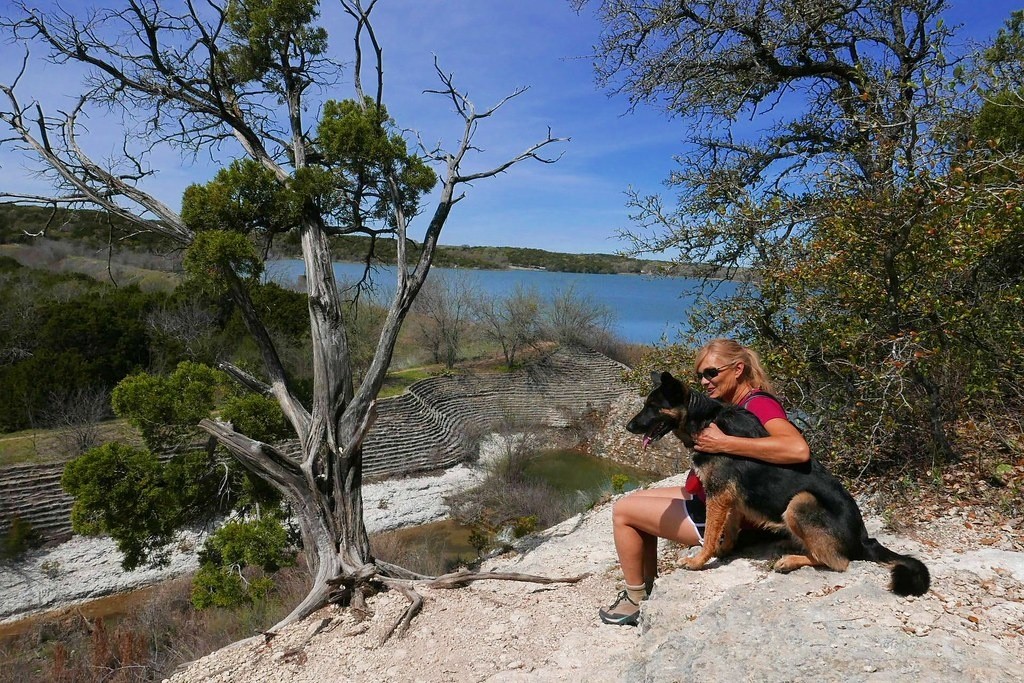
[626,370,930,597]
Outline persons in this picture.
[598,339,811,624]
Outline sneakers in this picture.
[598,584,647,626]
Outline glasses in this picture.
[691,361,738,382]
